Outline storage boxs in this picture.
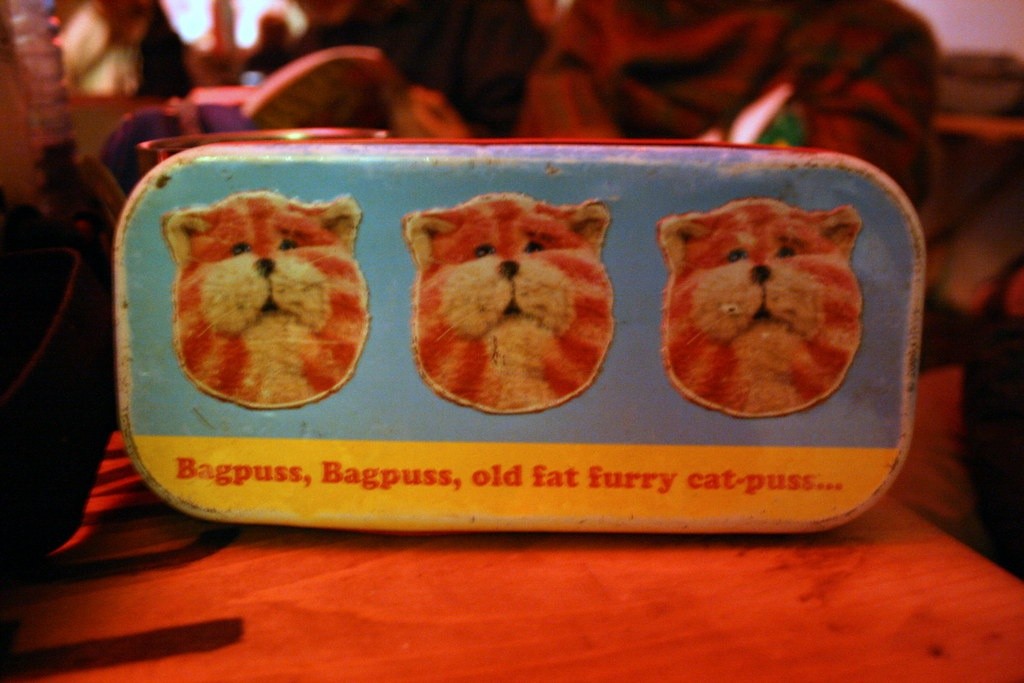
[115,138,926,532]
